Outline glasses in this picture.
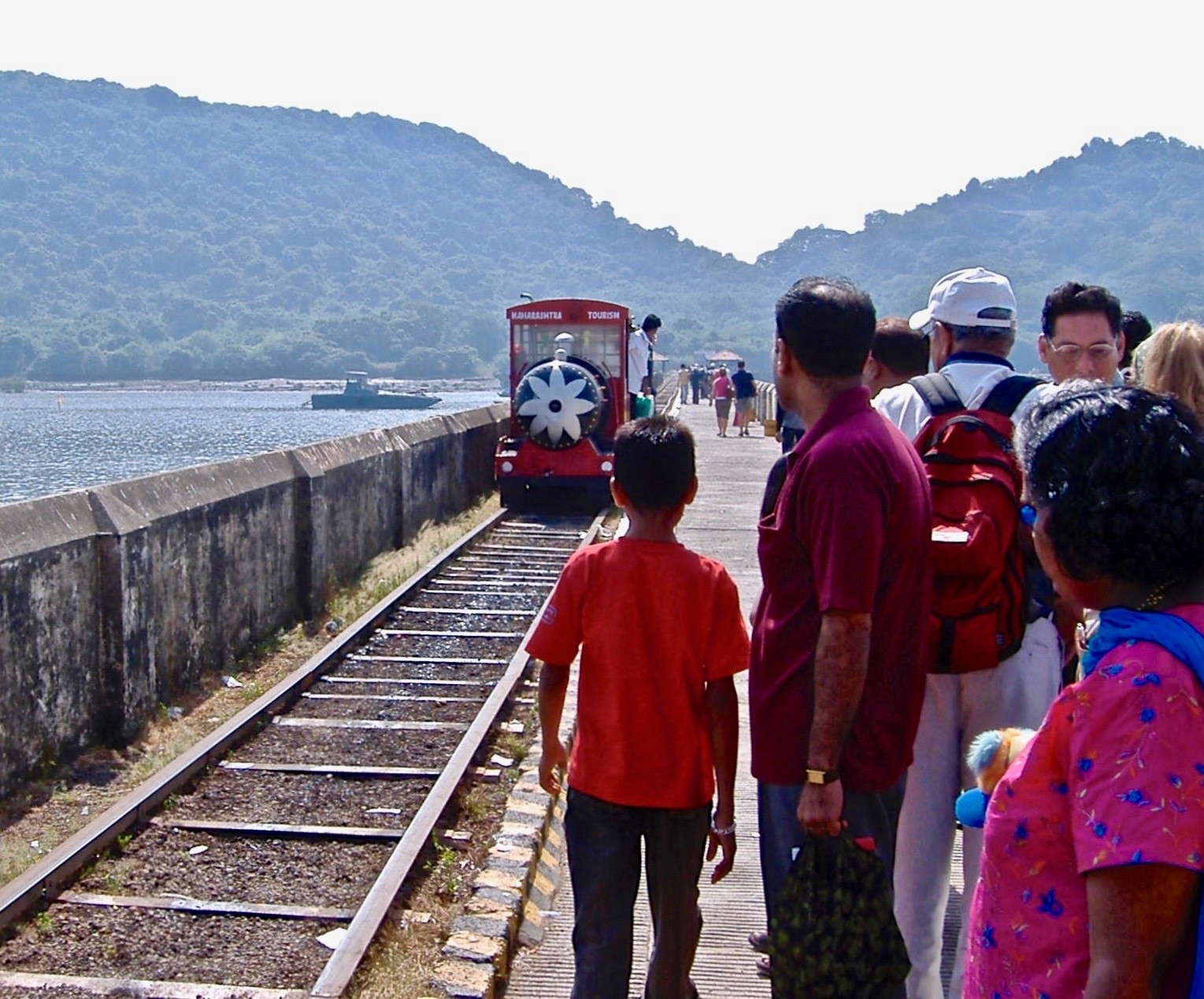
[1044,331,1119,362]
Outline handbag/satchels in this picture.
[767,823,911,999]
[633,391,656,421]
[724,384,735,400]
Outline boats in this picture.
[310,370,443,409]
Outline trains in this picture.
[493,291,654,506]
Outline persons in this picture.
[677,360,756,437]
[525,416,750,999]
[624,314,661,421]
[751,269,1203,999]
[961,380,1204,999]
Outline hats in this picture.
[907,265,1018,330]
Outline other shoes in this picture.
[744,431,749,435]
[723,434,727,437]
[684,402,687,404]
[681,402,683,405]
[755,955,770,978]
[717,433,722,436]
[738,433,743,437]
[748,929,769,952]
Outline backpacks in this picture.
[898,369,1051,676]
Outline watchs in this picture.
[803,766,841,785]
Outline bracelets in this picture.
[711,808,736,835]
